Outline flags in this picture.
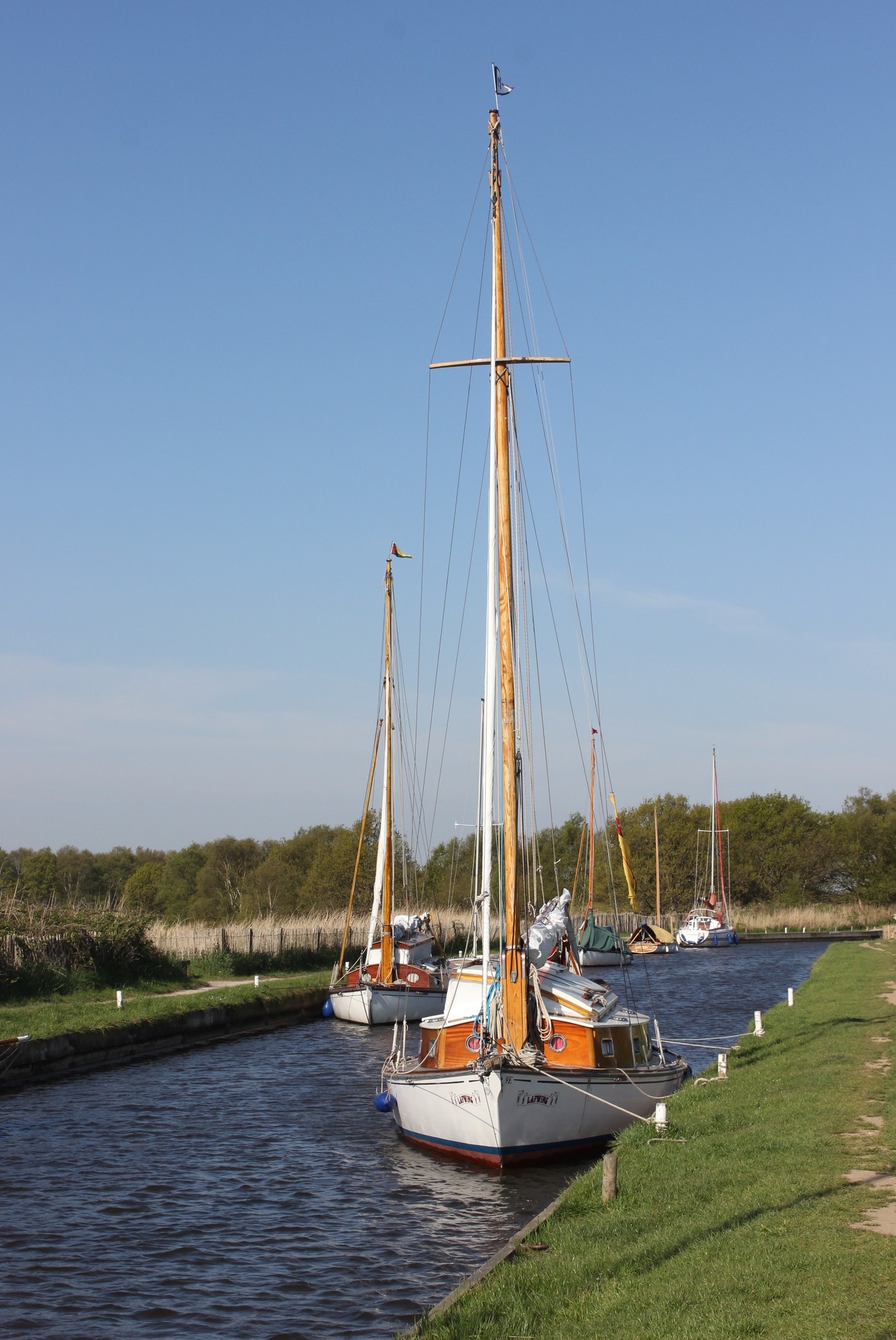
[493,64,515,96]
[609,791,637,912]
[390,541,414,558]
[592,728,598,735]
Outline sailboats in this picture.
[677,747,739,949]
[323,557,449,1025]
[563,739,633,967]
[373,106,692,1169]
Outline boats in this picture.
[626,922,678,954]
